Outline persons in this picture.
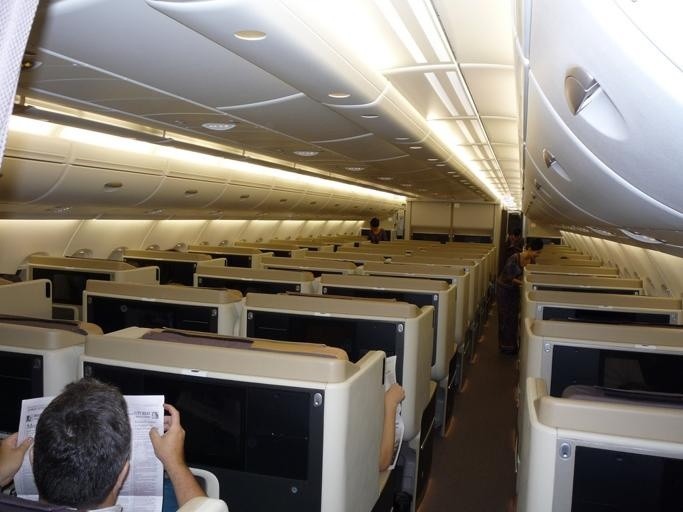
[504,228,524,265]
[494,238,544,349]
[0,374,229,512]
[367,217,388,244]
[379,384,406,473]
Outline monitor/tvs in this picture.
[1,347,47,437]
[193,273,303,298]
[531,284,641,296]
[540,338,683,411]
[50,304,78,322]
[186,241,623,287]
[25,264,113,308]
[241,306,405,416]
[320,283,440,367]
[534,303,678,330]
[82,290,219,336]
[77,355,325,511]
[120,255,199,289]
[552,438,683,512]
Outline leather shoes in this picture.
[502,349,518,356]
